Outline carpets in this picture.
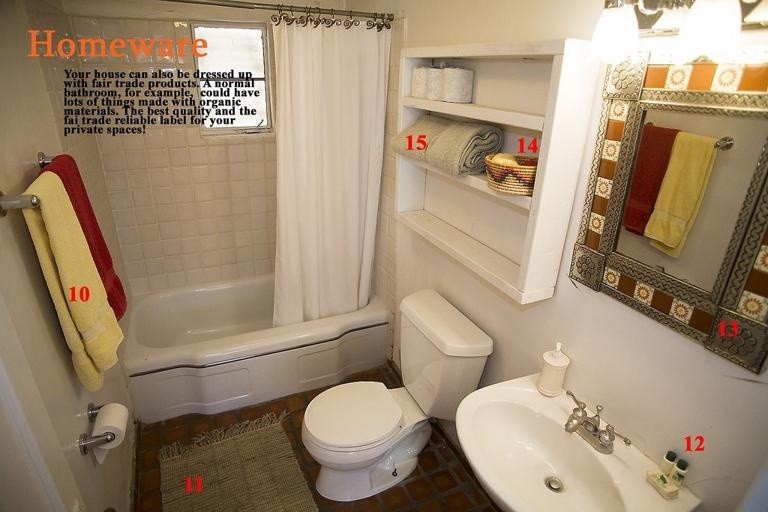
[158,410,318,512]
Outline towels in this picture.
[23,171,124,392]
[645,131,715,260]
[42,145,128,323]
[619,124,676,234]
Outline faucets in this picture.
[564,405,603,435]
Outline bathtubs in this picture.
[122,273,389,424]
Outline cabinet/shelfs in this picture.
[395,38,604,306]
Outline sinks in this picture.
[455,373,701,511]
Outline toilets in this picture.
[302,290,494,500]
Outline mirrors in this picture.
[615,110,766,294]
